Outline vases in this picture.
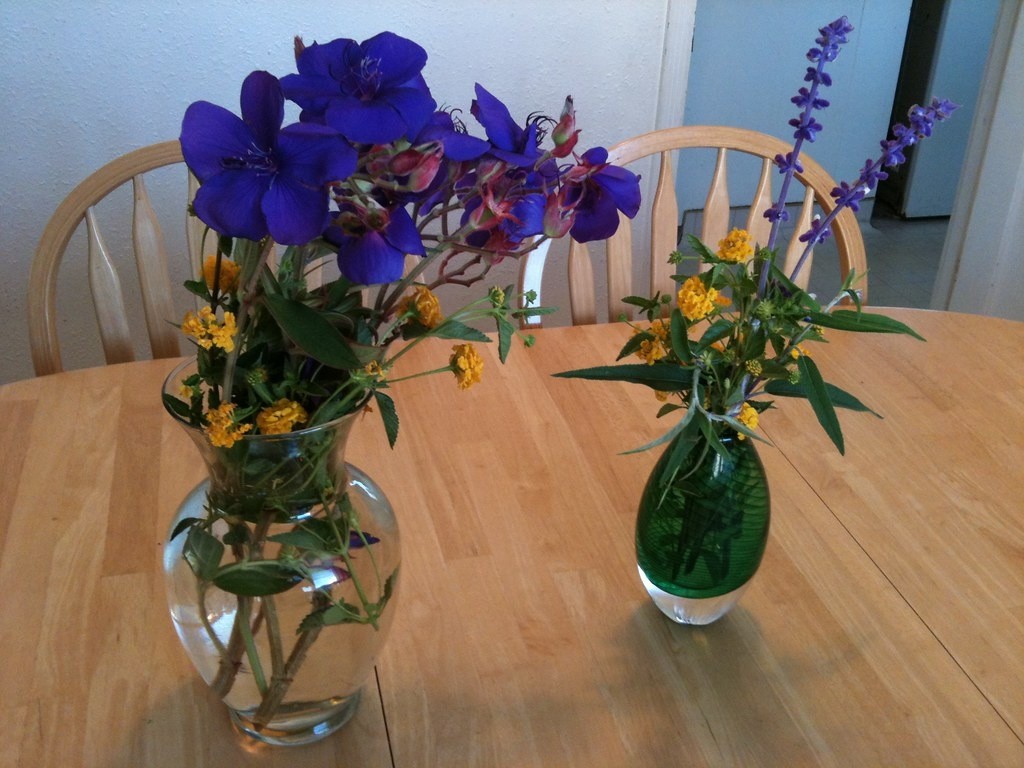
[160,434,403,753]
[633,397,774,636]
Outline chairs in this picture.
[518,104,869,327]
[24,126,430,377]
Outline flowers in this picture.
[167,42,642,445]
[548,19,952,466]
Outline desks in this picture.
[2,302,1023,766]
